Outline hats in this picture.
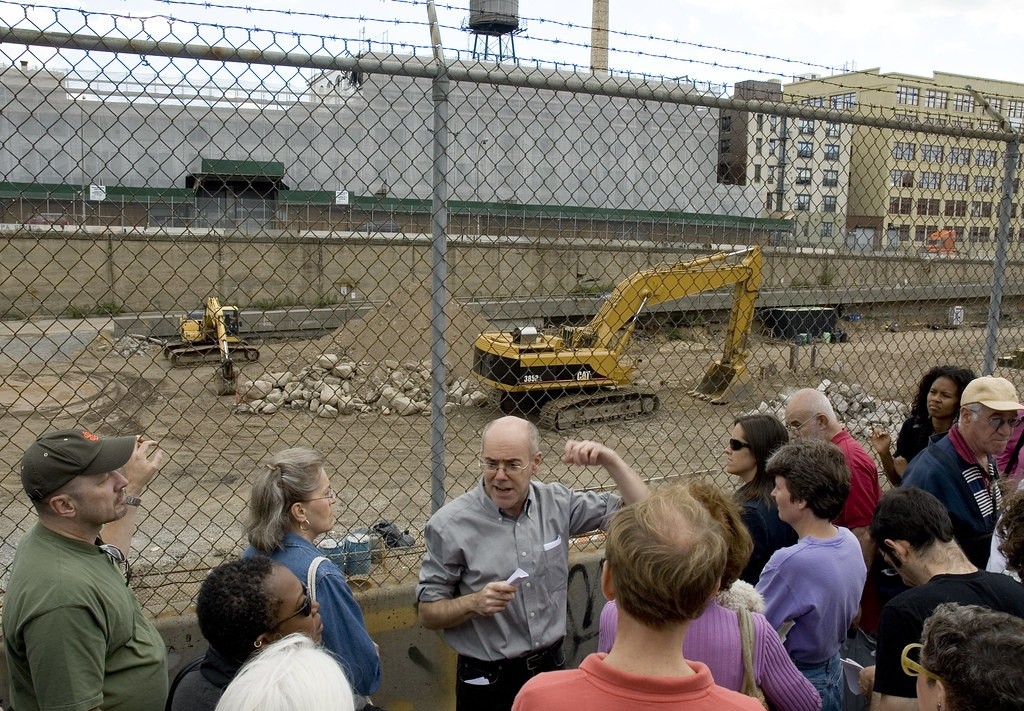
[21,430,136,500]
[960,375,1024,410]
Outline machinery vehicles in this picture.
[164,296,261,397]
[470,245,763,435]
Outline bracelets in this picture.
[125,495,141,506]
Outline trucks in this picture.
[919,229,957,254]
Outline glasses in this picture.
[730,438,751,450]
[478,450,535,474]
[285,487,334,513]
[786,412,821,436]
[880,550,898,576]
[969,408,1022,428]
[901,643,949,696]
[265,583,311,631]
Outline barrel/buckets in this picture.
[345,533,371,575]
[317,539,344,575]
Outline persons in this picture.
[510,484,766,711]
[598,479,823,711]
[901,375,1024,585]
[901,603,1024,711]
[2,429,169,711]
[416,415,652,711]
[754,439,868,711]
[172,556,323,711]
[214,632,354,711]
[869,487,1024,711]
[785,388,883,711]
[724,414,799,590]
[868,365,977,488]
[242,447,381,711]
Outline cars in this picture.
[355,220,400,233]
[21,212,70,225]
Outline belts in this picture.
[458,637,564,671]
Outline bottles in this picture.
[849,314,861,320]
[927,324,939,330]
[823,332,831,343]
[885,324,888,332]
[836,328,840,342]
[370,533,385,564]
